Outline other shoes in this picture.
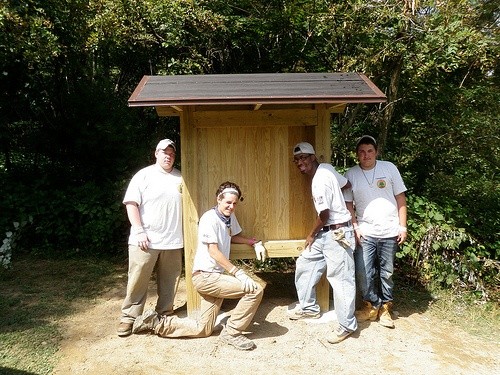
[116,323,133,336]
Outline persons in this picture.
[342,135,408,328]
[132,182,267,349]
[289,142,359,344]
[117,139,183,337]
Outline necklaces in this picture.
[360,161,376,188]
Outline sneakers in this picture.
[326,325,354,344]
[287,304,321,320]
[132,308,159,334]
[219,322,255,349]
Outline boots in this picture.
[356,297,382,322]
[378,301,395,328]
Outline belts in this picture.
[192,270,202,277]
[321,221,351,232]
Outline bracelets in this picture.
[134,226,145,234]
[399,226,407,233]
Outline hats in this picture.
[293,142,316,156]
[155,138,177,152]
[355,133,377,146]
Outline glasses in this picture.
[293,156,310,162]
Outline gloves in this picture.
[251,240,267,262]
[335,232,352,249]
[234,268,258,294]
[134,226,151,250]
